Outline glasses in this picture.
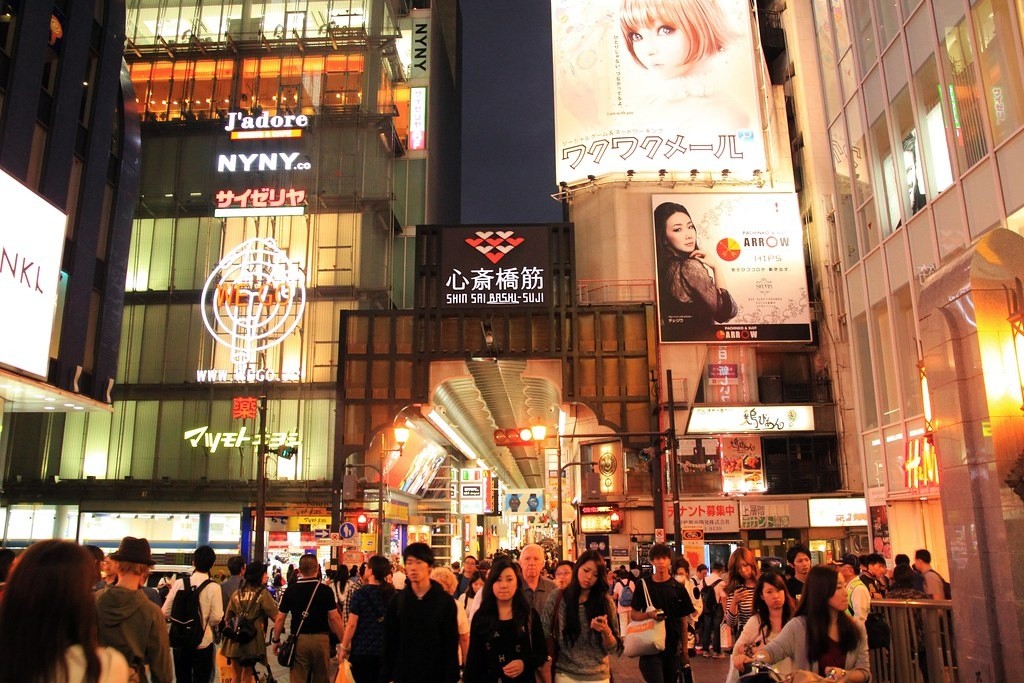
[364,563,369,568]
[555,570,572,575]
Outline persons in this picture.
[655,202,738,341]
[616,0,753,104]
[890,132,927,233]
[0,534,955,683]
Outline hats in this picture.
[834,553,860,568]
[110,537,157,565]
[476,560,491,570]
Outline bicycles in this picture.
[740,652,837,683]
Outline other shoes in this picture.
[712,652,726,658]
[703,650,713,657]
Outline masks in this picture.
[674,575,686,583]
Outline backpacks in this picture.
[618,579,633,606]
[225,588,262,645]
[701,579,722,616]
[168,577,215,649]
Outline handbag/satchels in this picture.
[534,656,553,683]
[679,665,695,683]
[930,570,950,600]
[720,619,733,650]
[334,658,355,683]
[865,612,890,649]
[545,633,556,661]
[624,578,666,658]
[278,634,298,667]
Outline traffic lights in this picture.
[493,427,533,447]
[611,510,621,530]
[355,514,366,533]
[276,444,295,459]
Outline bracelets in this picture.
[601,630,612,639]
[272,638,280,643]
[339,643,349,651]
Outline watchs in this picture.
[527,494,539,511]
[508,495,521,512]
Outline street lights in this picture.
[620,489,681,544]
[530,424,599,565]
[343,418,410,557]
